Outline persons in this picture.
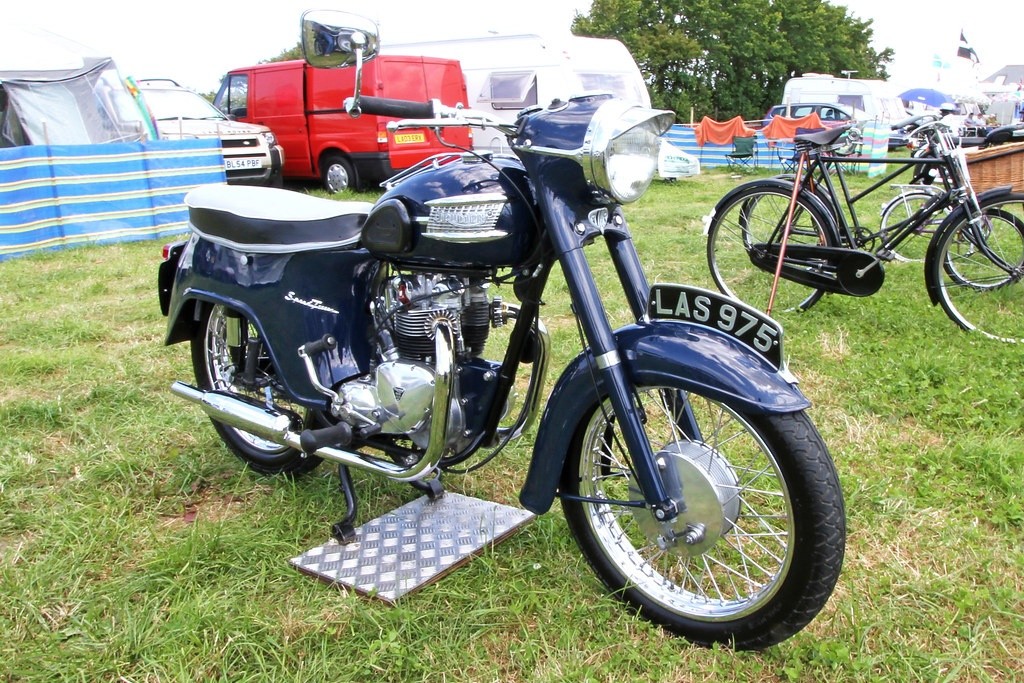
[963,113,993,136]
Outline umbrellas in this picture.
[898,89,955,110]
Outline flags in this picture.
[958,35,980,64]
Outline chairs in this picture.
[725,135,757,173]
[955,140,1024,253]
[777,142,865,175]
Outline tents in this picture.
[979,65,1024,91]
[0,27,157,146]
